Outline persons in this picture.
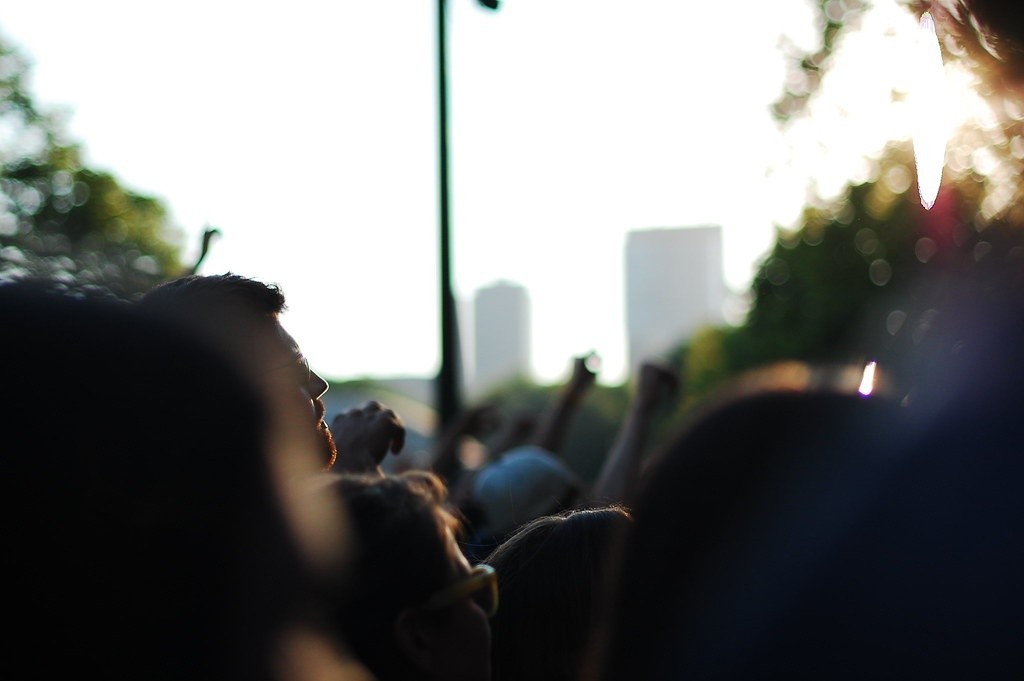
[0,261,1021,681]
